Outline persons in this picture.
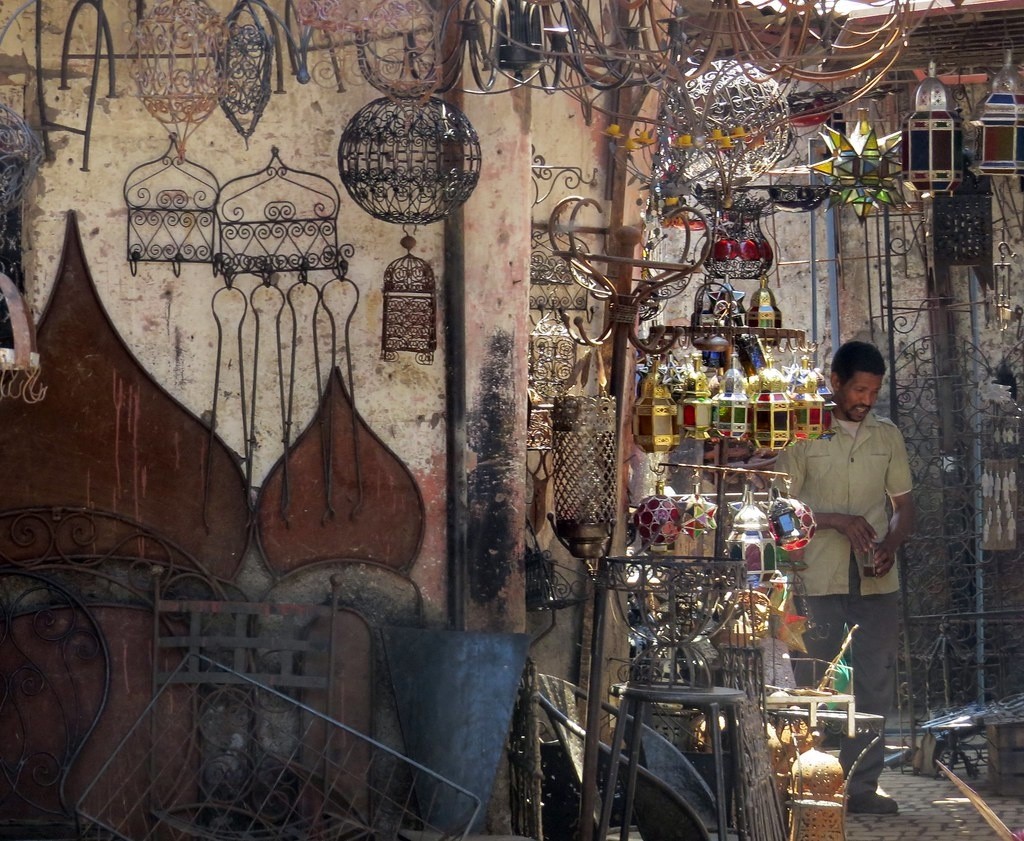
[769,340,914,815]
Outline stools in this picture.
[598,682,748,841]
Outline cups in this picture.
[864,539,883,577]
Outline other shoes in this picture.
[847,792,898,813]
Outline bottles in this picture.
[732,315,767,382]
